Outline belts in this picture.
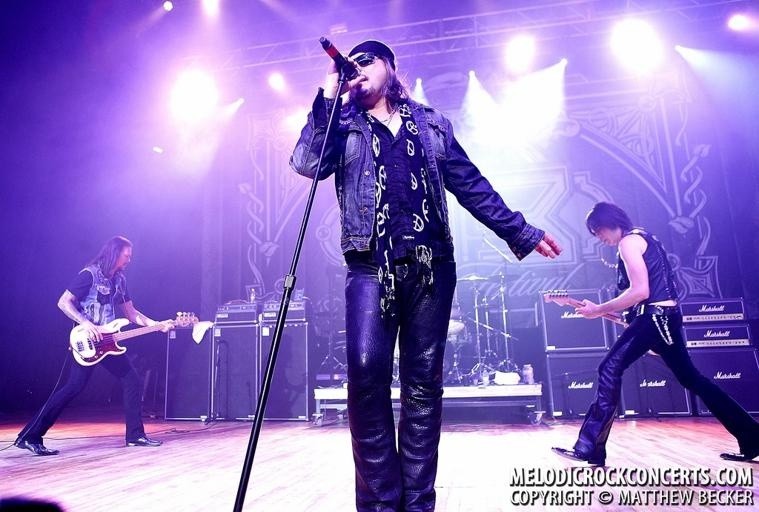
[633,304,684,317]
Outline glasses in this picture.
[353,51,377,65]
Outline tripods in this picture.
[205,338,236,425]
[313,273,349,387]
[444,281,538,387]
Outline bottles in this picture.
[523,363,534,385]
[250,288,255,304]
[483,368,490,385]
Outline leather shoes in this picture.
[14,438,59,455]
[125,437,162,446]
[719,449,758,461]
[550,445,605,467]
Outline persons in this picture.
[11,236,175,455]
[550,201,759,470]
[288,38,562,512]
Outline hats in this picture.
[347,40,394,61]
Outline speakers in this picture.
[618,350,693,418]
[539,289,609,353]
[687,348,759,417]
[545,352,618,419]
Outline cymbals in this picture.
[473,305,496,308]
[488,275,519,283]
[457,275,488,281]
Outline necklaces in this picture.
[374,102,401,129]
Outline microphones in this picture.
[320,37,358,80]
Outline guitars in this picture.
[543,290,661,357]
[70,311,199,367]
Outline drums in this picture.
[448,305,465,334]
